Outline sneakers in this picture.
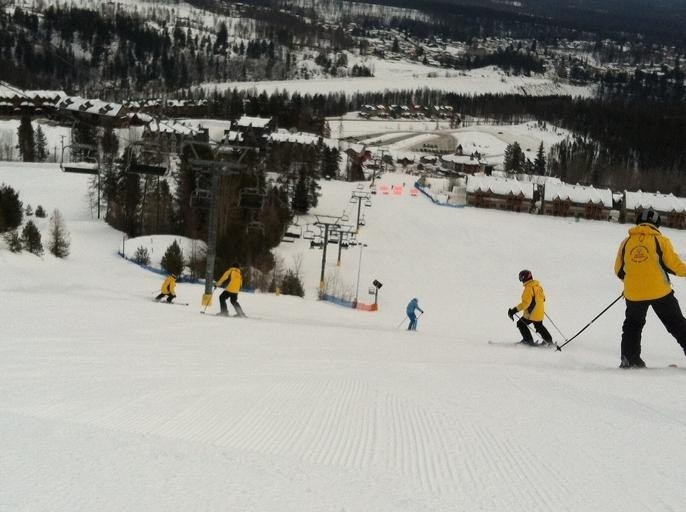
[216,312,245,317]
[621,360,645,368]
[521,338,551,346]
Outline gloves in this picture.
[508,308,517,318]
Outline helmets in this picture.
[171,271,177,279]
[232,262,240,268]
[519,270,532,283]
[636,210,661,228]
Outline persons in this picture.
[614,209,686,371]
[406,297,423,331]
[507,270,553,345]
[154,270,177,303]
[214,262,245,316]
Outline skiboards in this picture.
[488,338,558,350]
[148,297,190,305]
[205,311,253,318]
